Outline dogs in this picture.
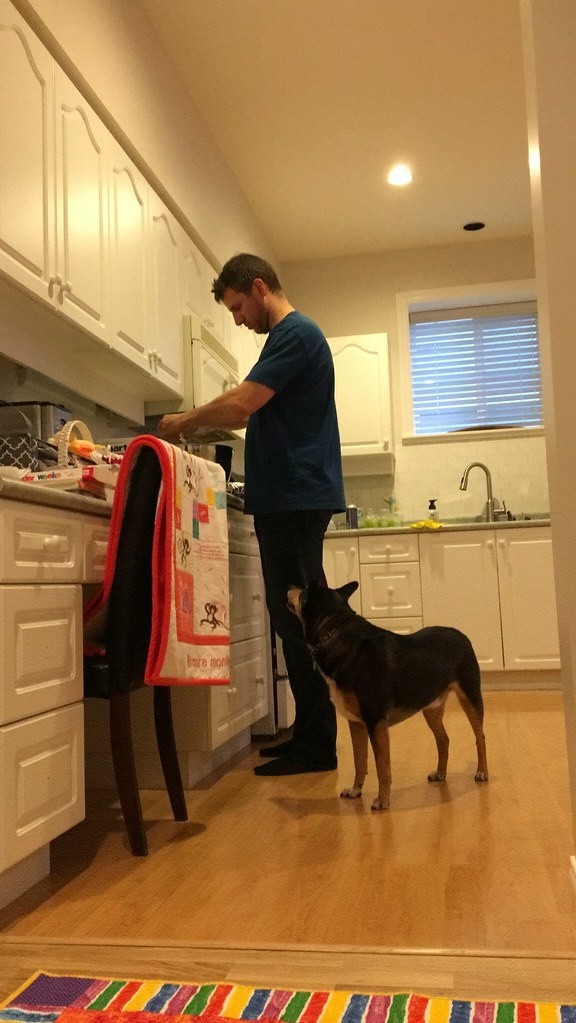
[285,582,489,811]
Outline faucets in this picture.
[459,461,506,522]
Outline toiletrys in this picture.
[427,499,440,524]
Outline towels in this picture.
[410,519,446,530]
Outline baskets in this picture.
[41,420,95,470]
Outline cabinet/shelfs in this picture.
[1,0,266,430]
[321,527,561,692]
[0,495,279,908]
[327,333,396,478]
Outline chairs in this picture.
[83,445,188,857]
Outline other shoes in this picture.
[254,749,338,776]
[259,737,296,758]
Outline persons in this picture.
[155,253,345,777]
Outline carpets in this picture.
[0,970,576,1023]
